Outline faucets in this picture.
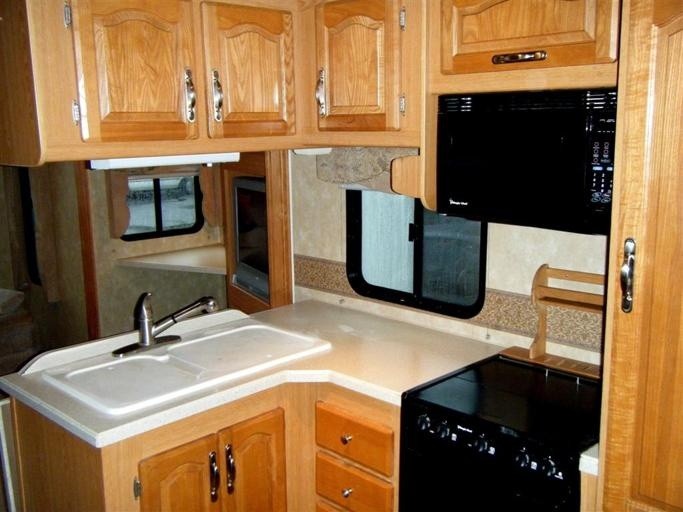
[137,290,219,347]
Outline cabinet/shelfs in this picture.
[426,0,622,96]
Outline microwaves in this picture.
[432,90,619,239]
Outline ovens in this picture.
[397,351,604,511]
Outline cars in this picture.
[126,176,192,197]
